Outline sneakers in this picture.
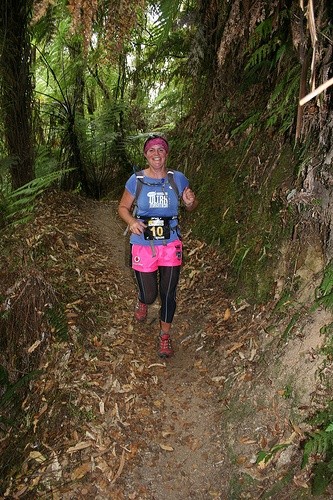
[135,299,147,321]
[158,333,173,357]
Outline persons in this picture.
[116,134,199,358]
